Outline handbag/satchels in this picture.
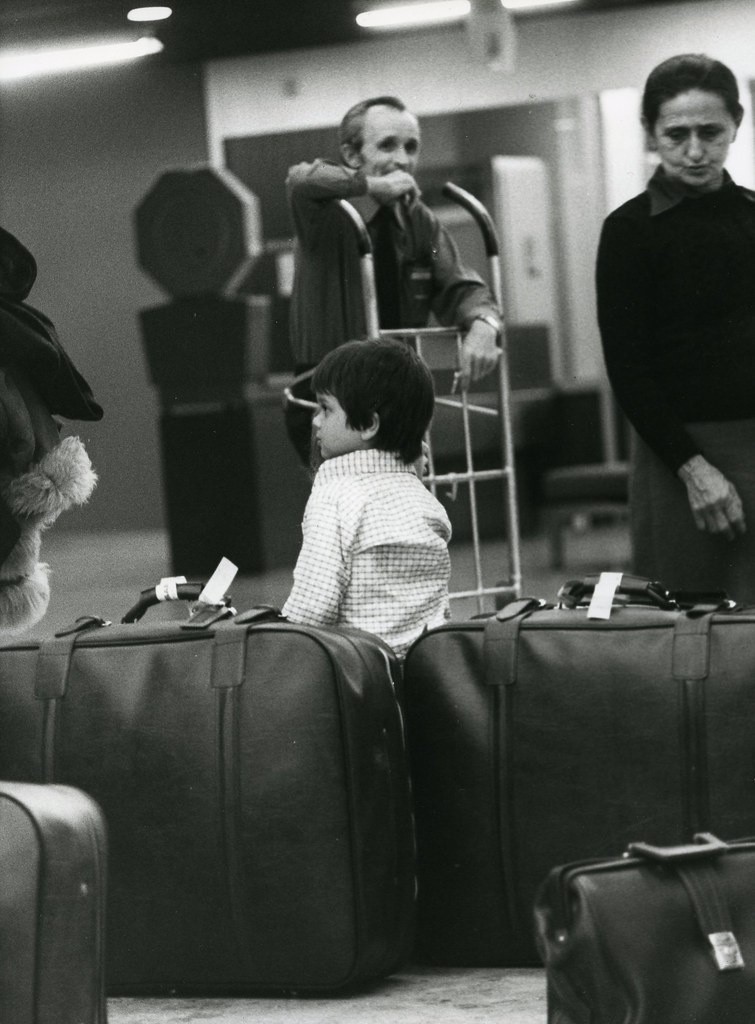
[532,832,755,1024]
[0,584,415,998]
[0,783,108,1024]
[398,576,755,970]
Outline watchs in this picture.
[475,314,502,334]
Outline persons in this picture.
[596,54,755,607]
[284,95,504,485]
[282,336,453,678]
